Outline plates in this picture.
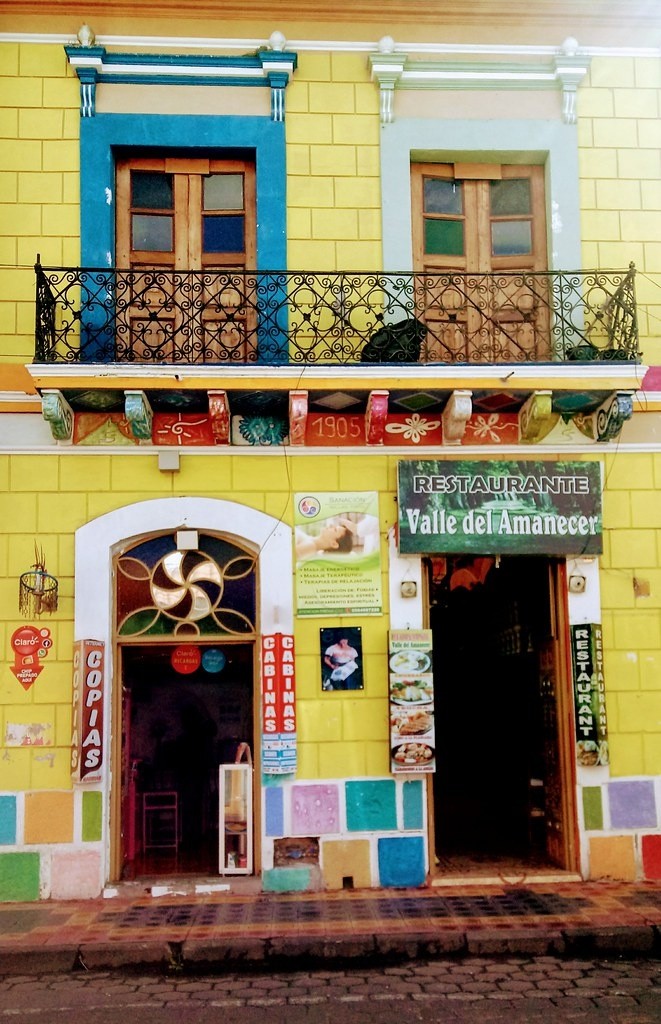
[576,740,599,766]
[392,743,435,766]
[390,691,433,706]
[600,741,610,767]
[389,650,431,674]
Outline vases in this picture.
[565,346,598,360]
[598,350,627,360]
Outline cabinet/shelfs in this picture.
[218,763,253,877]
[143,792,177,857]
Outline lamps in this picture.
[16,563,57,616]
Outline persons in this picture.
[325,633,358,690]
[295,524,351,558]
[341,512,379,552]
